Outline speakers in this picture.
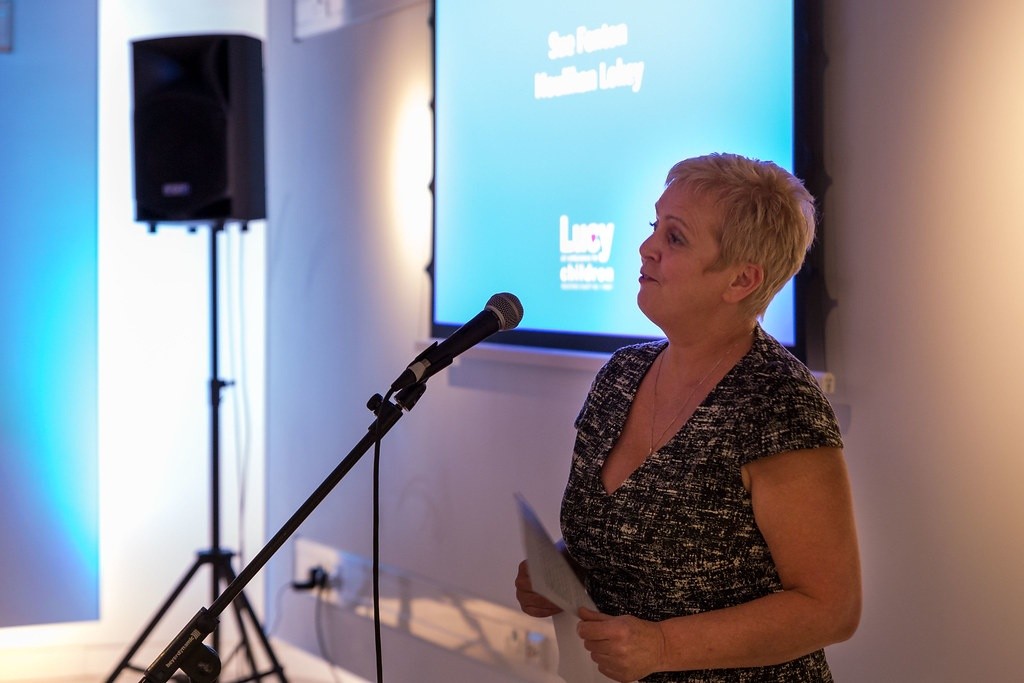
[132,33,267,228]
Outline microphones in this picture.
[391,292,524,395]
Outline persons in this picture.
[515,152,864,683]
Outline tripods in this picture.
[103,227,289,683]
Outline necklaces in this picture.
[644,328,756,452]
